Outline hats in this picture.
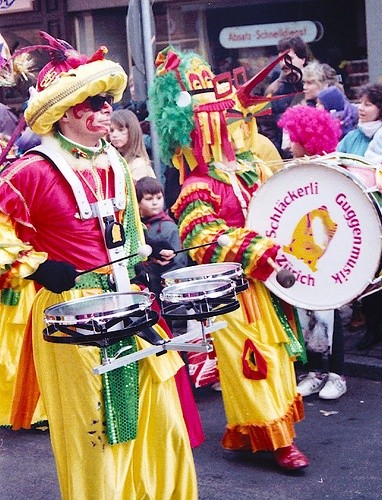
[1,31,129,136]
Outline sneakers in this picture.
[296,370,329,396]
[318,371,347,400]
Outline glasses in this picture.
[88,94,115,112]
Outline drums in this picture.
[42,290,159,349]
[160,261,249,293]
[159,278,240,320]
[244,151,382,311]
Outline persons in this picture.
[0,30,200,499]
[0,32,382,470]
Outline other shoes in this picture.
[273,444,310,471]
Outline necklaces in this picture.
[73,160,111,200]
